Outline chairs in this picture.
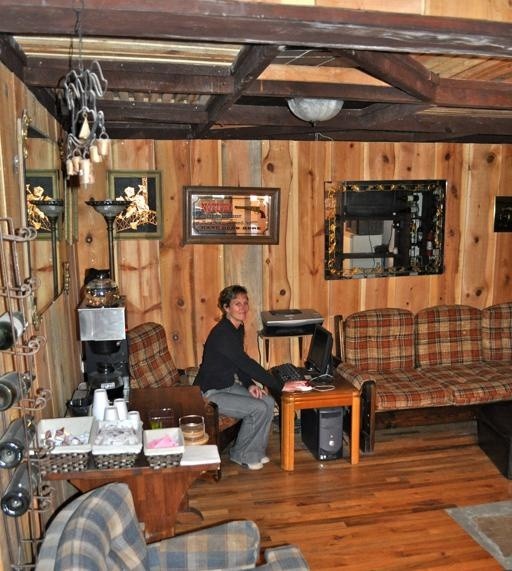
[35,481,311,571]
[127,322,264,449]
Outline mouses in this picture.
[297,386,313,392]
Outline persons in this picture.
[193,284,309,472]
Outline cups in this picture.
[92,388,141,422]
[177,414,206,443]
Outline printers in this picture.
[260,308,324,336]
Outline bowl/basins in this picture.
[66,398,89,417]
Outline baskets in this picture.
[27,416,185,475]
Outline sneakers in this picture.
[229,456,270,471]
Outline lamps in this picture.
[29,199,65,298]
[287,96,344,126]
[84,200,132,282]
[56,0,111,184]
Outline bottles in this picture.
[0,455,39,517]
[0,414,36,471]
[0,311,27,351]
[0,369,37,412]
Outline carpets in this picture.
[444,500,512,571]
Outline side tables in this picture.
[267,368,361,471]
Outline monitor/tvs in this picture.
[344,190,408,217]
[306,325,333,374]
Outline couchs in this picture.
[334,302,512,453]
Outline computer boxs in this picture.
[300,407,344,461]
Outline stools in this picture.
[477,400,512,479]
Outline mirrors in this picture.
[324,178,448,280]
[16,109,71,331]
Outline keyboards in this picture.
[270,362,305,387]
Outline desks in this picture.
[257,324,322,370]
[41,386,221,545]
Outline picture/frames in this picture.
[183,186,280,245]
[26,169,60,241]
[493,195,512,232]
[106,169,163,239]
